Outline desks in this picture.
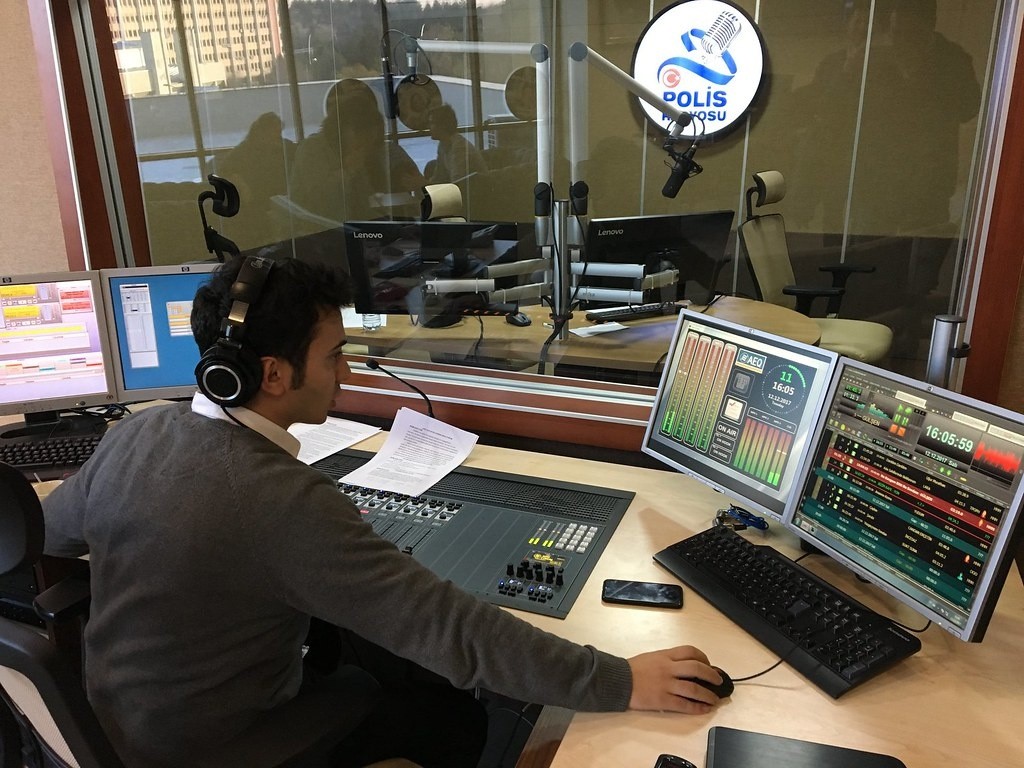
[344,295,821,385]
[0,400,1024,768]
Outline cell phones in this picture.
[602,579,683,609]
[654,755,697,768]
[676,666,734,706]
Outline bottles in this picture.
[362,314,381,333]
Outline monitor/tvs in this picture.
[0,269,117,445]
[343,221,518,328]
[99,262,225,404]
[781,357,1024,642]
[639,308,840,526]
[576,210,735,312]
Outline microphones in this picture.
[366,359,433,417]
[380,39,397,119]
[662,131,704,199]
[698,12,740,61]
[405,38,418,80]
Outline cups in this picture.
[33,480,63,501]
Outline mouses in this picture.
[505,312,531,326]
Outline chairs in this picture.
[739,169,892,363]
[421,184,468,224]
[0,461,125,768]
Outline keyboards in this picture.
[0,436,103,482]
[652,523,922,700]
[586,303,688,324]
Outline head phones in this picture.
[194,255,275,407]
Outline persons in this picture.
[38,262,724,768]
[219,98,491,226]
